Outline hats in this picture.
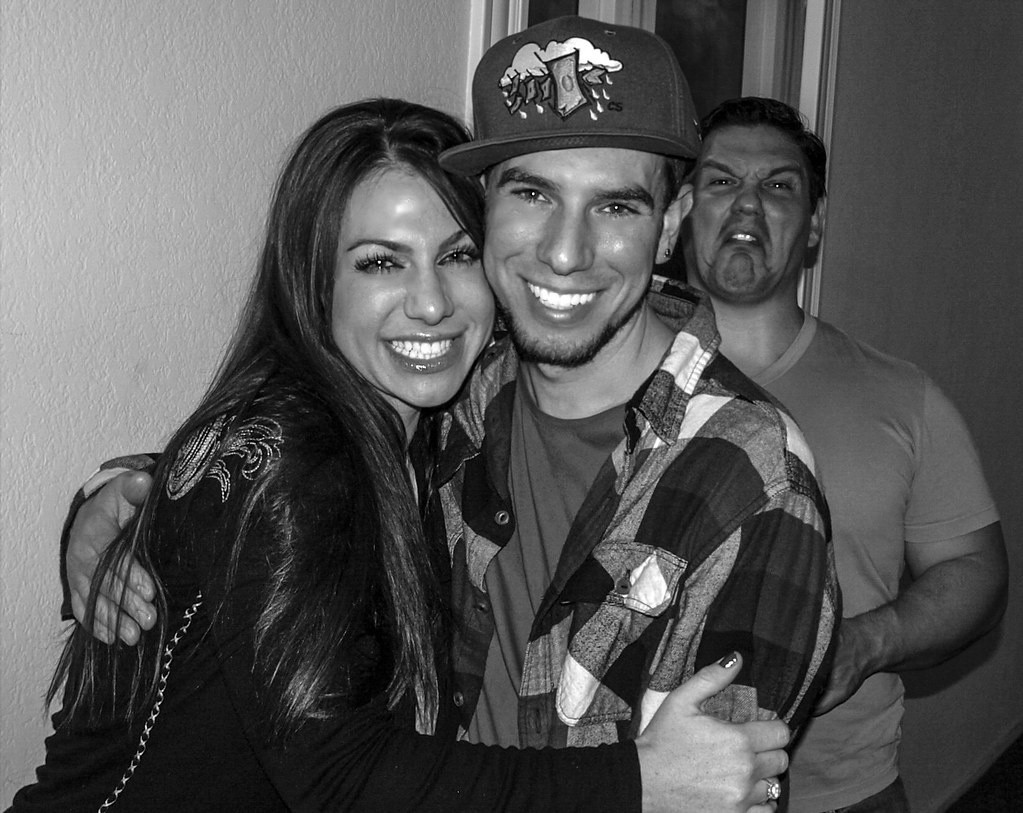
[436,17,708,177]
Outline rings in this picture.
[758,777,781,807]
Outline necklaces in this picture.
[525,360,540,409]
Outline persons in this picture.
[59,14,846,812]
[677,94,1010,812]
[1,95,791,812]
